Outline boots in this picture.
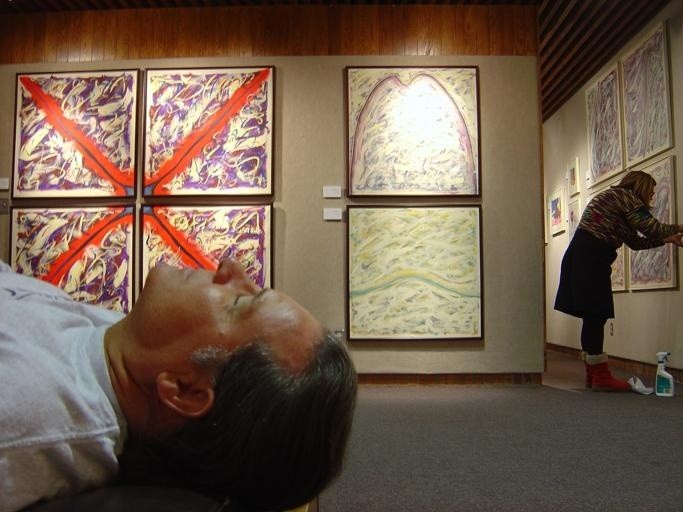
[581,351,632,392]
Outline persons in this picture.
[0,256,358,512]
[554,171,683,393]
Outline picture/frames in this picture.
[9,69,141,200]
[342,65,485,343]
[585,16,677,292]
[143,65,275,201]
[140,201,275,299]
[548,156,581,242]
[9,203,137,312]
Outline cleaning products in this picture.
[655,352,675,398]
